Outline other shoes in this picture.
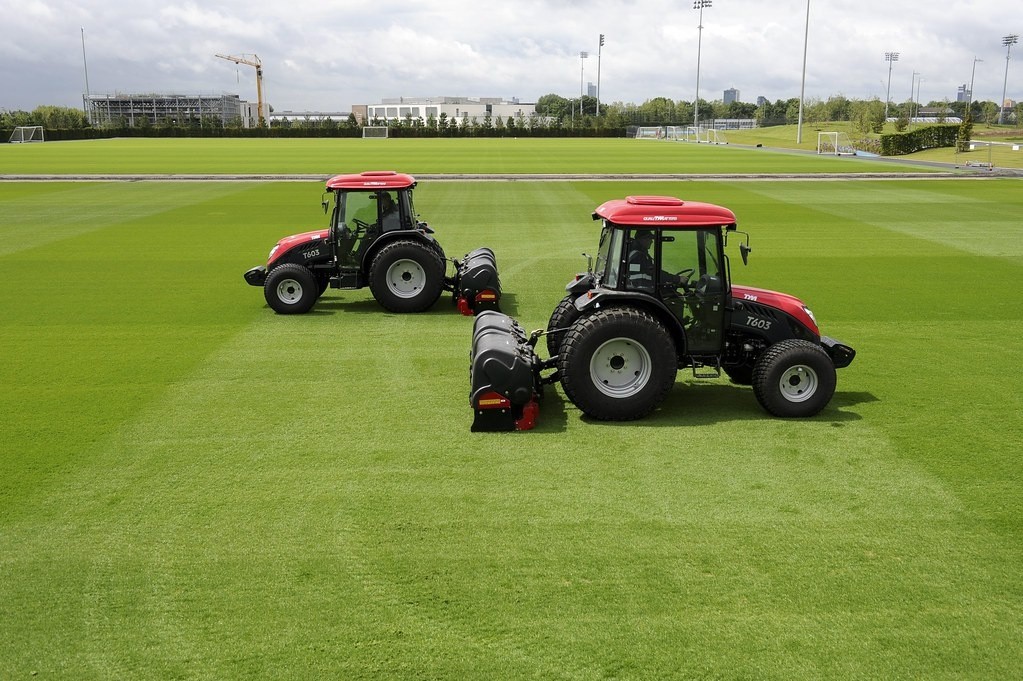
[347,255,359,265]
[682,315,689,325]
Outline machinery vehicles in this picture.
[243,172,503,316]
[470,197,855,431]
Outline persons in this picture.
[629,230,690,324]
[352,193,398,255]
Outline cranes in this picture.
[214,52,263,127]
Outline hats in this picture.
[634,229,655,242]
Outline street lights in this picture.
[885,51,900,120]
[693,0,713,133]
[997,35,1018,124]
[969,57,983,109]
[909,69,926,124]
[595,33,605,115]
